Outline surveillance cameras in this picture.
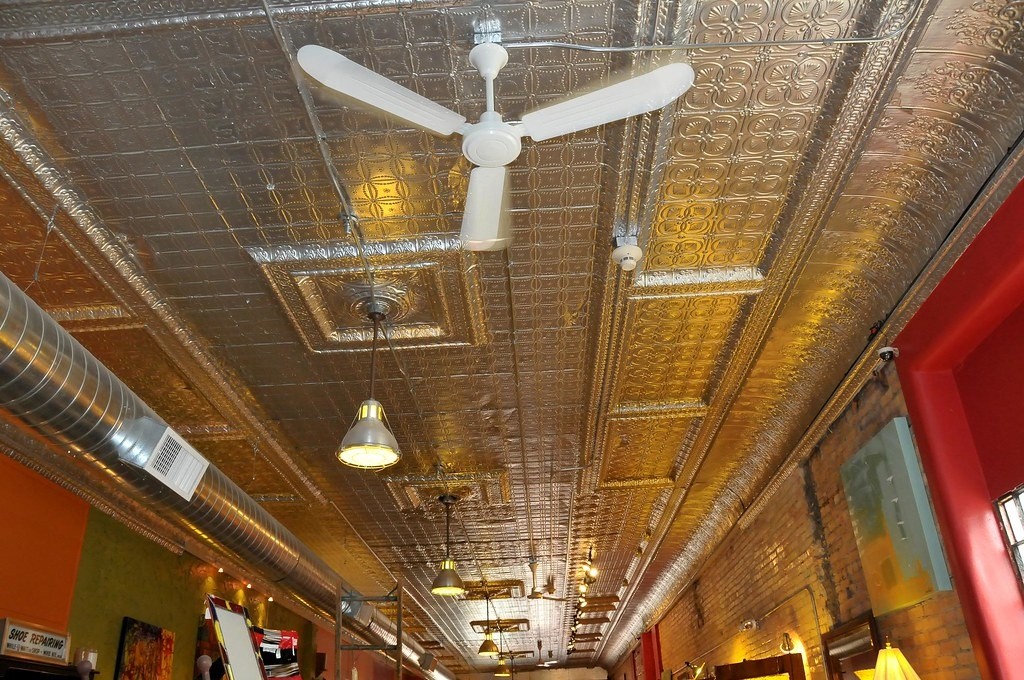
[877,347,894,361]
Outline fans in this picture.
[517,645,559,668]
[504,562,568,602]
[296,44,695,250]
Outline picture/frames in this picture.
[205,593,269,680]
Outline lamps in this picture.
[567,557,593,655]
[780,633,794,653]
[873,635,922,680]
[335,313,402,469]
[477,594,500,657]
[684,662,705,680]
[492,626,512,676]
[431,496,466,596]
